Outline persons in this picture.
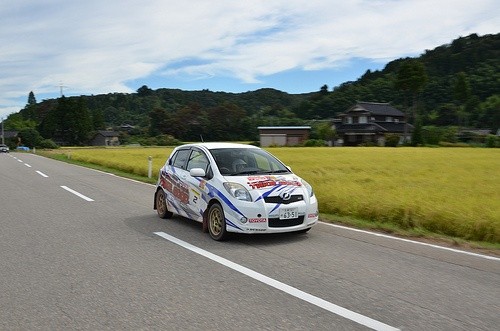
[232,159,247,174]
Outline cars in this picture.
[0,144,10,153]
[153,142,319,241]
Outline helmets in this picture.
[232,159,247,172]
